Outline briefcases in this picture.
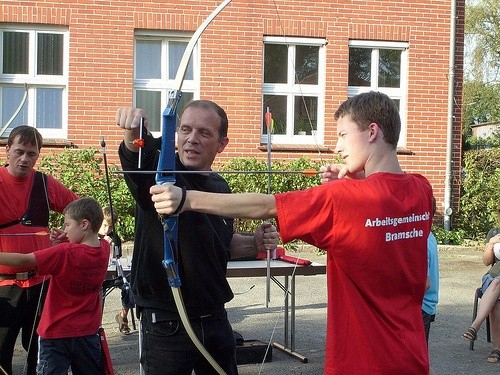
[233,340,273,365]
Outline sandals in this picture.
[115,312,131,335]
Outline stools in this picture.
[469,287,491,350]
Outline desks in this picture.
[105,258,327,375]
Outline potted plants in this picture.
[295,115,317,136]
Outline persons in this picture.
[150,91,433,375]
[0,197,105,375]
[421,197,440,343]
[114,100,280,375]
[0,126,81,375]
[98,206,137,334]
[462,208,500,364]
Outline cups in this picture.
[127,248,132,266]
